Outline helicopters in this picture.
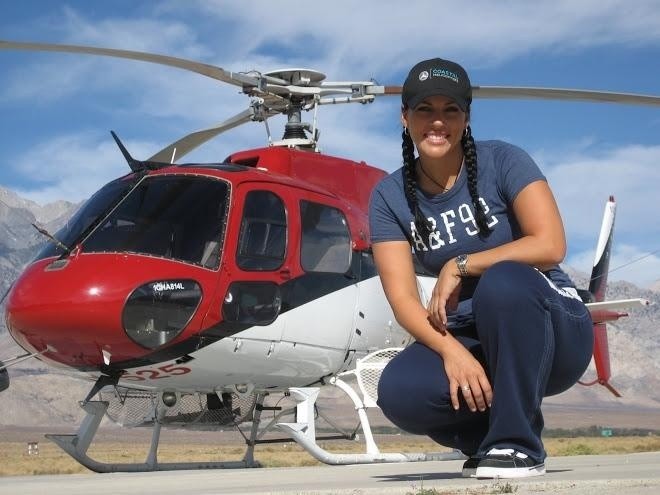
[0,41,660,474]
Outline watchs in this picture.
[454,252,470,280]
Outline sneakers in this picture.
[462,448,546,478]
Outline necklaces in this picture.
[418,156,465,191]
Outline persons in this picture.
[368,58,594,479]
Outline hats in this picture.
[402,59,472,112]
